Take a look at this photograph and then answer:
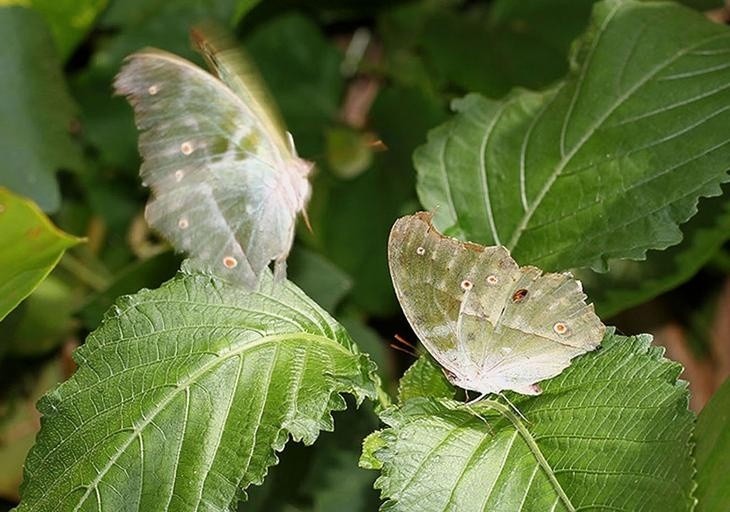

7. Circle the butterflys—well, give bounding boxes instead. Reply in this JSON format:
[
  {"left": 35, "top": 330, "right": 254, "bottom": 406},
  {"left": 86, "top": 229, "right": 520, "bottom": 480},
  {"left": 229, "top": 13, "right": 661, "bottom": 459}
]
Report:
[
  {"left": 109, "top": 19, "right": 316, "bottom": 295},
  {"left": 389, "top": 204, "right": 607, "bottom": 437}
]
